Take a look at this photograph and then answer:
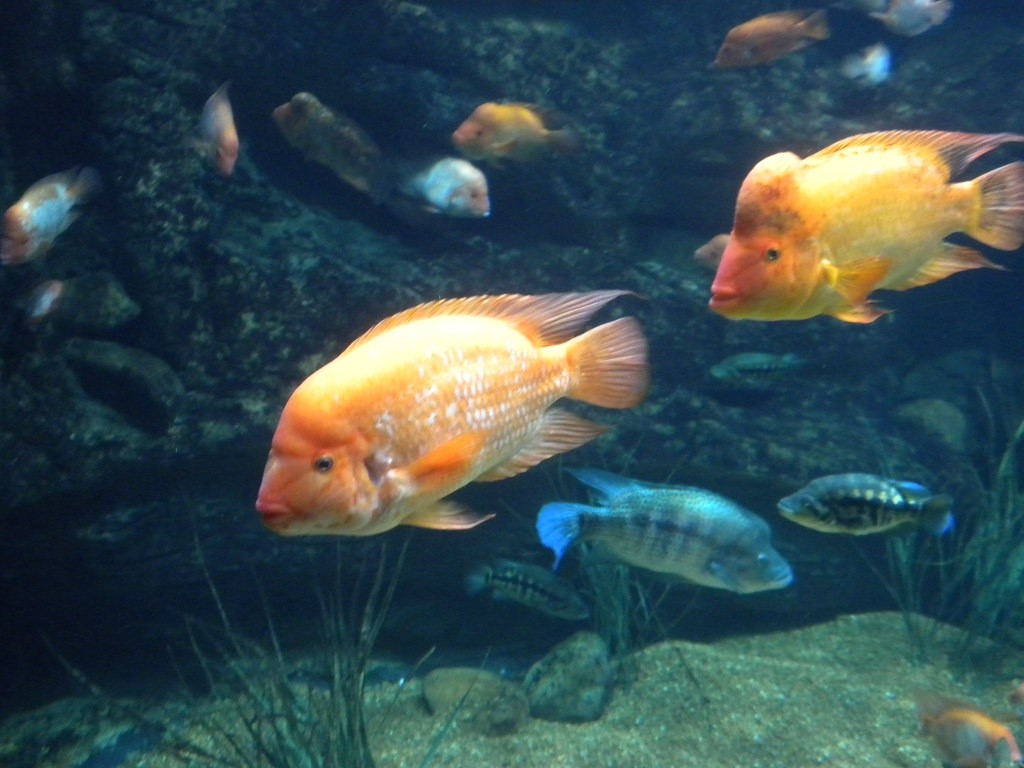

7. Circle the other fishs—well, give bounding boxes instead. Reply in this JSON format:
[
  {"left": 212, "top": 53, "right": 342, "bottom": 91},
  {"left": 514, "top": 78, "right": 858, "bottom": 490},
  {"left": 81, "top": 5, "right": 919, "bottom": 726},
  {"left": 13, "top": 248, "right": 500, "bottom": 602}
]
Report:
[{"left": 0, "top": 1, "right": 1023, "bottom": 768}]
